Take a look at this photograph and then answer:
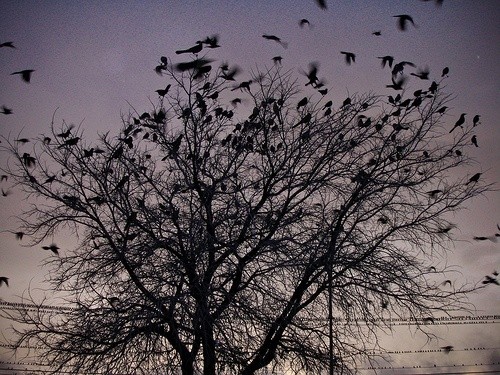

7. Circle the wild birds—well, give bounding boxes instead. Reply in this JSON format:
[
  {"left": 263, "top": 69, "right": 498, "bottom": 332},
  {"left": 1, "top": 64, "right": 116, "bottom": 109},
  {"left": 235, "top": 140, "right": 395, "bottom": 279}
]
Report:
[
  {"left": 105, "top": 1, "right": 500, "bottom": 356},
  {"left": 0, "top": 298, "right": 499, "bottom": 375},
  {"left": 1, "top": 40, "right": 102, "bottom": 288}
]
